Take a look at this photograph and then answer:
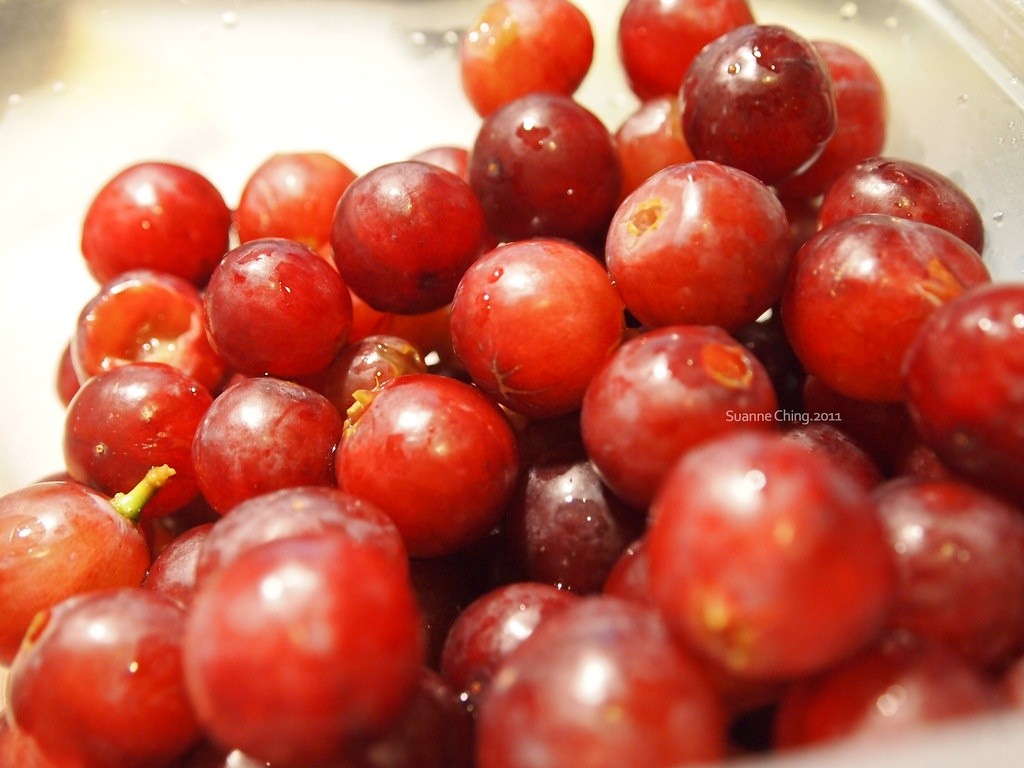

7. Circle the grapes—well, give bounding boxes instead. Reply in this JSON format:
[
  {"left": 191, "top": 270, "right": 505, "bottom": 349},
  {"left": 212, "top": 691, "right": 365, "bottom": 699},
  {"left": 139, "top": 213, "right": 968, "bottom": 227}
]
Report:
[{"left": 0, "top": 0, "right": 1024, "bottom": 768}]
